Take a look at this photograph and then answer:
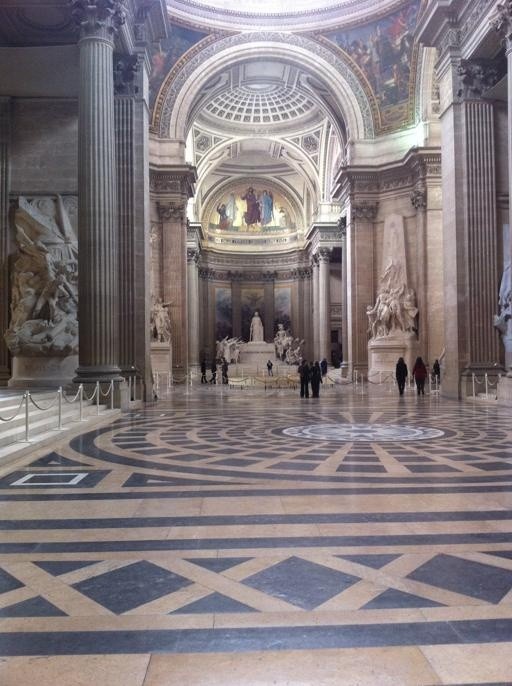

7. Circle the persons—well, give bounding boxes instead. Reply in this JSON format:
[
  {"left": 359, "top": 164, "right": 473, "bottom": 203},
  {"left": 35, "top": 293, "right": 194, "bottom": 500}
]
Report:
[
  {"left": 220, "top": 357, "right": 229, "bottom": 384},
  {"left": 366, "top": 282, "right": 419, "bottom": 340},
  {"left": 298, "top": 358, "right": 327, "bottom": 400},
  {"left": 216, "top": 336, "right": 228, "bottom": 358},
  {"left": 200, "top": 358, "right": 208, "bottom": 383},
  {"left": 223, "top": 341, "right": 235, "bottom": 361},
  {"left": 273, "top": 324, "right": 305, "bottom": 365},
  {"left": 250, "top": 311, "right": 262, "bottom": 341},
  {"left": 267, "top": 359, "right": 273, "bottom": 377},
  {"left": 433, "top": 359, "right": 439, "bottom": 378},
  {"left": 216, "top": 186, "right": 274, "bottom": 230},
  {"left": 396, "top": 357, "right": 408, "bottom": 395},
  {"left": 209, "top": 358, "right": 217, "bottom": 384},
  {"left": 412, "top": 357, "right": 427, "bottom": 396},
  {"left": 152, "top": 297, "right": 173, "bottom": 342}
]
